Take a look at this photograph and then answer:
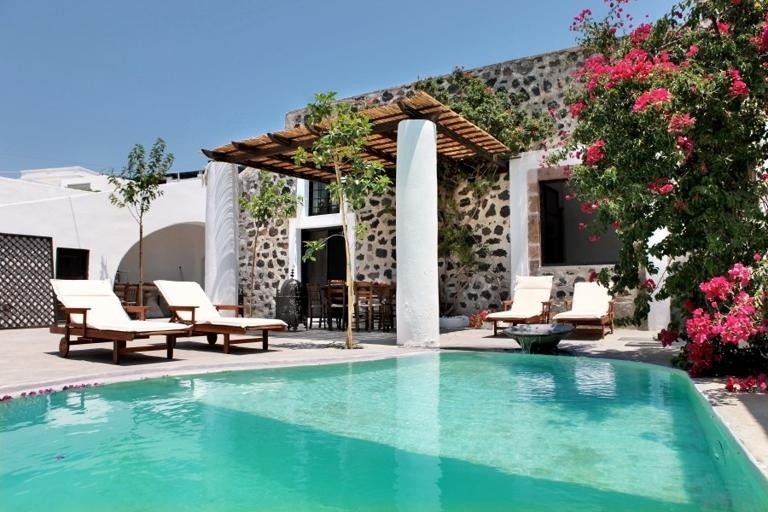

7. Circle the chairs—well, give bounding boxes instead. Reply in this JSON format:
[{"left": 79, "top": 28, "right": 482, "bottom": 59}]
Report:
[
  {"left": 305, "top": 280, "right": 396, "bottom": 332},
  {"left": 485, "top": 275, "right": 554, "bottom": 336},
  {"left": 121, "top": 282, "right": 139, "bottom": 305},
  {"left": 50, "top": 278, "right": 194, "bottom": 364},
  {"left": 152, "top": 280, "right": 289, "bottom": 353},
  {"left": 552, "top": 282, "right": 618, "bottom": 339}
]
